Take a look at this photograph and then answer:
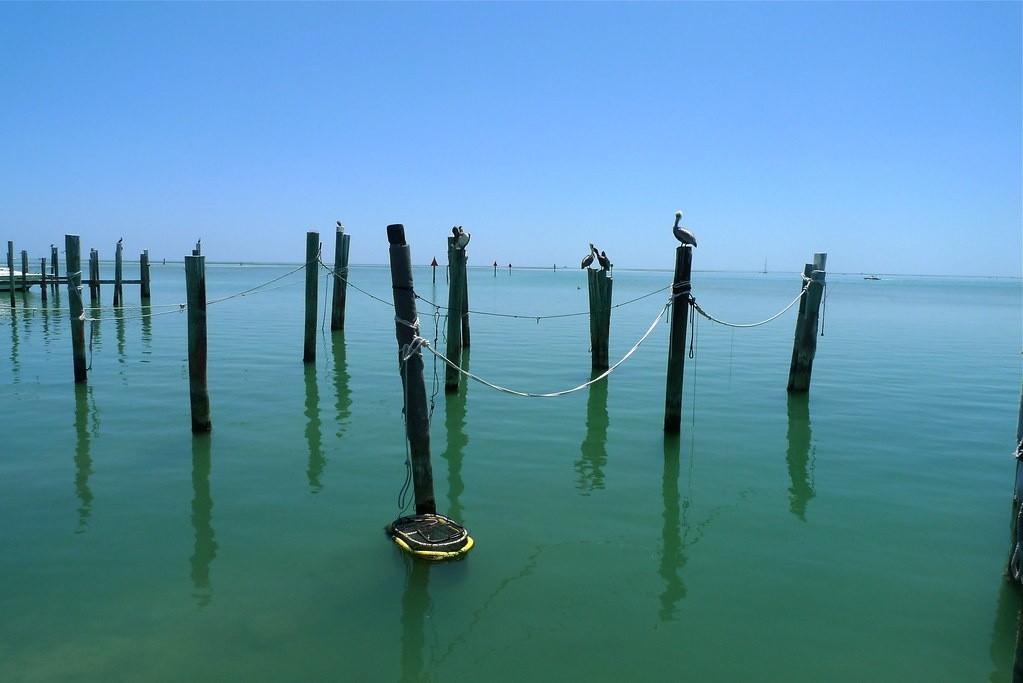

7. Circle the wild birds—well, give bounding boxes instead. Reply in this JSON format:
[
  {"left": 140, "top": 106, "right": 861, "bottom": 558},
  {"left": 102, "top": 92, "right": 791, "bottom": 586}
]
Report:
[
  {"left": 118, "top": 237, "right": 123, "bottom": 243},
  {"left": 449, "top": 226, "right": 471, "bottom": 249},
  {"left": 580, "top": 243, "right": 610, "bottom": 271},
  {"left": 179, "top": 304, "right": 186, "bottom": 309},
  {"left": 197, "top": 237, "right": 201, "bottom": 244},
  {"left": 672, "top": 211, "right": 697, "bottom": 247}
]
